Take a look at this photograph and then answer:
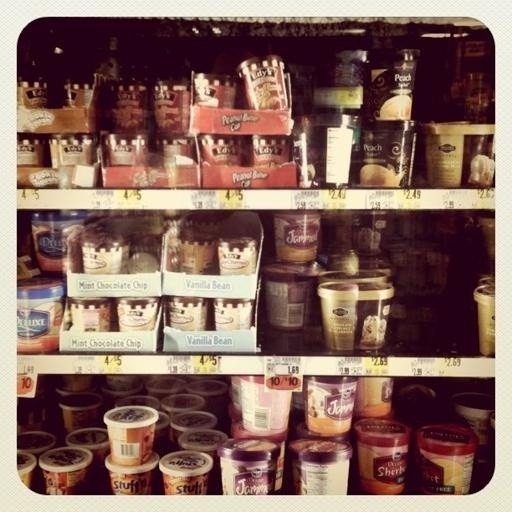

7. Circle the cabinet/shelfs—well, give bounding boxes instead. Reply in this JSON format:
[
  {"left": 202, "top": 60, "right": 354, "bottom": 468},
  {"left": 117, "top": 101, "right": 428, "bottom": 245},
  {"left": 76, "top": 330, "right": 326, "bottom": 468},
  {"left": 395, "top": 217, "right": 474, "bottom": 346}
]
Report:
[{"left": 17, "top": 187, "right": 496, "bottom": 379}]
